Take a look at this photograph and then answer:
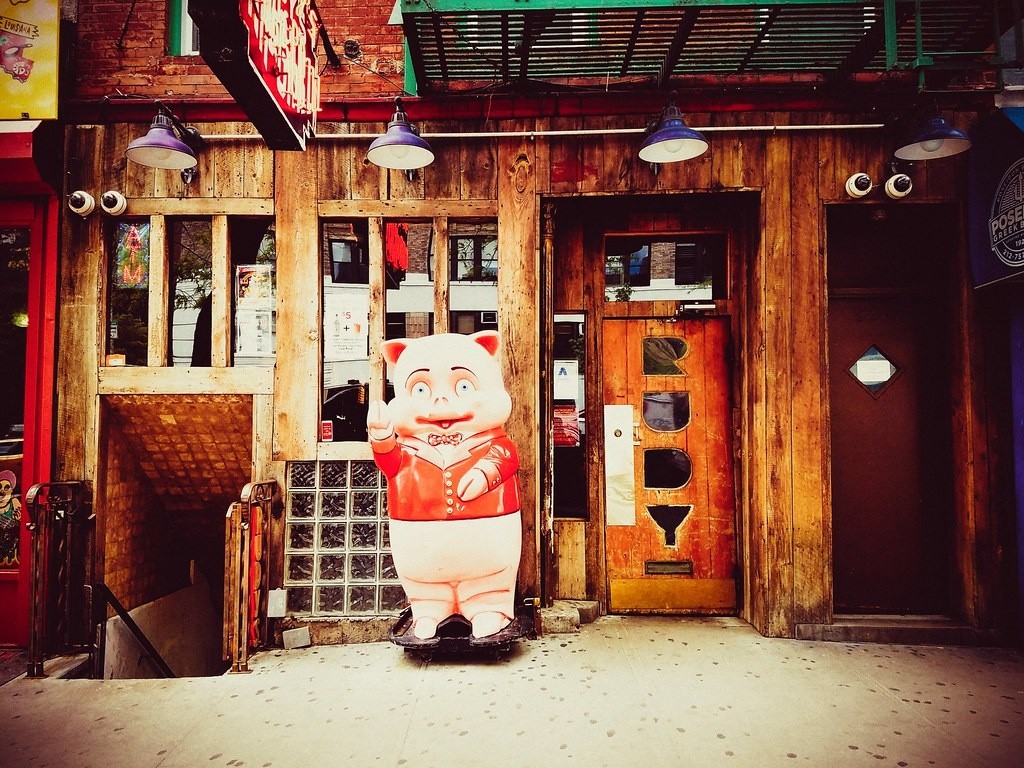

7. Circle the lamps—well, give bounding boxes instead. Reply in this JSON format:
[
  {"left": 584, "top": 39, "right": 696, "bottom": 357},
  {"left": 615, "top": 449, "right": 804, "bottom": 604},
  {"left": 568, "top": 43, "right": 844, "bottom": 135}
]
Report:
[
  {"left": 124, "top": 96, "right": 200, "bottom": 185},
  {"left": 366, "top": 96, "right": 434, "bottom": 180},
  {"left": 638, "top": 91, "right": 709, "bottom": 175},
  {"left": 886, "top": 95, "right": 973, "bottom": 185}
]
